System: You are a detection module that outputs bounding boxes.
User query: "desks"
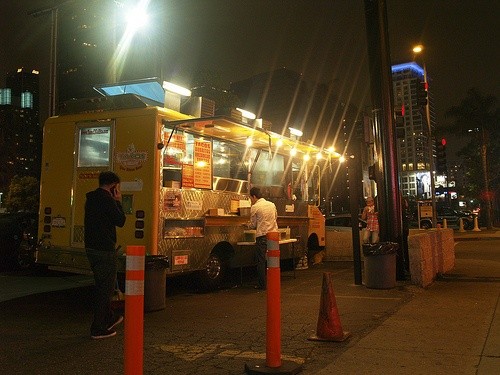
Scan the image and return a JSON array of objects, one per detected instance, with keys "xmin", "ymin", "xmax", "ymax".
[{"xmin": 237, "ymin": 237, "xmax": 298, "ymax": 289}]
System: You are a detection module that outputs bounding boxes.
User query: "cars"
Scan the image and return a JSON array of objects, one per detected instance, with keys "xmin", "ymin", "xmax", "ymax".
[{"xmin": 420, "ymin": 206, "xmax": 481, "ymax": 230}]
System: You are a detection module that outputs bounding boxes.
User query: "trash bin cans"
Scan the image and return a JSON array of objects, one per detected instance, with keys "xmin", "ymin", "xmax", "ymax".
[
  {"xmin": 118, "ymin": 254, "xmax": 169, "ymax": 312},
  {"xmin": 360, "ymin": 243, "xmax": 399, "ymax": 289}
]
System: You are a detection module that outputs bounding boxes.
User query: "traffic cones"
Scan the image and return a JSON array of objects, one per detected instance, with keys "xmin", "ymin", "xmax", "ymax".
[{"xmin": 306, "ymin": 271, "xmax": 352, "ymax": 342}]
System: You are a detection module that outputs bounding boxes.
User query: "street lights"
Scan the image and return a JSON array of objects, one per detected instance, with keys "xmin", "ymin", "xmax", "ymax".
[{"xmin": 404, "ymin": 44, "xmax": 424, "ymax": 230}]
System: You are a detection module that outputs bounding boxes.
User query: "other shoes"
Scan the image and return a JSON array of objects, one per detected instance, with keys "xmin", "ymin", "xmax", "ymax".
[
  {"xmin": 90, "ymin": 329, "xmax": 117, "ymax": 340},
  {"xmin": 107, "ymin": 314, "xmax": 124, "ymax": 331}
]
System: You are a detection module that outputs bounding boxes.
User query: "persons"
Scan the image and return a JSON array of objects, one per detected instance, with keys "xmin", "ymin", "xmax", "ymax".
[
  {"xmin": 360, "ymin": 198, "xmax": 379, "ymax": 243},
  {"xmin": 85, "ymin": 171, "xmax": 126, "ymax": 339},
  {"xmin": 242, "ymin": 187, "xmax": 279, "ymax": 290},
  {"xmin": 292, "ymin": 189, "xmax": 310, "ymax": 270}
]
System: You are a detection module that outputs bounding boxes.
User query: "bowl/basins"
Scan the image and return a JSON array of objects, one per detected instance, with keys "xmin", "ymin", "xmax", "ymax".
[
  {"xmin": 238, "ymin": 207, "xmax": 251, "ymax": 216},
  {"xmin": 244, "ymin": 230, "xmax": 256, "ymax": 242}
]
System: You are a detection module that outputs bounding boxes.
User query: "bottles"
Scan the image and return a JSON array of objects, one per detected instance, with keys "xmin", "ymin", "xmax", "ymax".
[{"xmin": 286, "ymin": 226, "xmax": 290, "ymax": 240}]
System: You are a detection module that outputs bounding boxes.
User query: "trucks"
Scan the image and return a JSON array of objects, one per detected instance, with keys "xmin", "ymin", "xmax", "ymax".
[{"xmin": 31, "ymin": 75, "xmax": 343, "ymax": 294}]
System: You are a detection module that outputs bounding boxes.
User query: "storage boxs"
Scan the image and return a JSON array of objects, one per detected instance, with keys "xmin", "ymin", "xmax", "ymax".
[
  {"xmin": 239, "ymin": 208, "xmax": 251, "ymax": 217},
  {"xmin": 244, "ymin": 230, "xmax": 256, "ymax": 242}
]
